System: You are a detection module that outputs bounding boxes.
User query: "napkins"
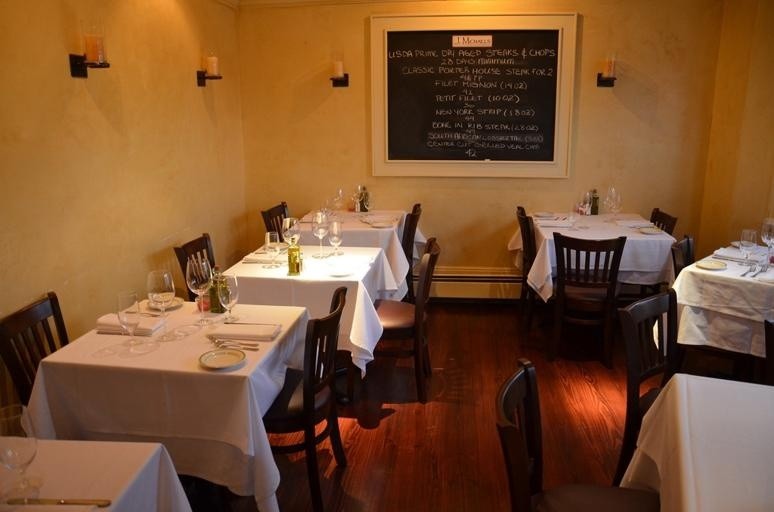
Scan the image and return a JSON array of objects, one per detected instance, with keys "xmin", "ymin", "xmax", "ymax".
[
  {"xmin": 616, "ymin": 221, "xmax": 650, "ymax": 228},
  {"xmin": 539, "ymin": 220, "xmax": 570, "ymax": 227},
  {"xmin": 362, "ymin": 215, "xmax": 388, "ymax": 223},
  {"xmin": 712, "ymin": 248, "xmax": 759, "ymax": 264},
  {"xmin": 97, "ymin": 313, "xmax": 164, "ymax": 336},
  {"xmin": 206, "ymin": 323, "xmax": 281, "ymax": 341},
  {"xmin": 242, "ymin": 254, "xmax": 274, "ymax": 264},
  {"xmin": 300, "ymin": 217, "xmax": 312, "ymax": 223}
]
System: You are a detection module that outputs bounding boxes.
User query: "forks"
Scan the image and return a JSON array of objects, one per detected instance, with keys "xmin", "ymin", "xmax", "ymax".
[
  {"xmin": 206, "ymin": 333, "xmax": 260, "ymax": 352},
  {"xmin": 740, "ymin": 264, "xmax": 768, "ymax": 279}
]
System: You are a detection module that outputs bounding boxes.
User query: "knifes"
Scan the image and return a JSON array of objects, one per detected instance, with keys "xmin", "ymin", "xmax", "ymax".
[{"xmin": 7, "ymin": 498, "xmax": 110, "ymax": 507}]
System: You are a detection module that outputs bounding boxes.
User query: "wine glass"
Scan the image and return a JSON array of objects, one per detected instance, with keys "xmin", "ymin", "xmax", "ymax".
[
  {"xmin": 740, "ymin": 219, "xmax": 774, "ymax": 268},
  {"xmin": 260, "ymin": 210, "xmax": 342, "ymax": 267},
  {"xmin": 115, "ymin": 291, "xmax": 145, "ymax": 347},
  {"xmin": 0, "ymin": 405, "xmax": 39, "ymax": 504},
  {"xmin": 603, "ymin": 187, "xmax": 623, "ymax": 224},
  {"xmin": 186, "ymin": 258, "xmax": 240, "ymax": 327},
  {"xmin": 324, "ymin": 186, "xmax": 376, "ymax": 222},
  {"xmin": 147, "ymin": 269, "xmax": 180, "ymax": 341}
]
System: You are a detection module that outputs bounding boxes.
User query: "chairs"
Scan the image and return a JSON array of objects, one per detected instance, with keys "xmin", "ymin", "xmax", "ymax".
[
  {"xmin": 611, "ymin": 288, "xmax": 682, "ymax": 487},
  {"xmin": 516, "ymin": 206, "xmax": 538, "ymax": 332},
  {"xmin": 261, "ymin": 202, "xmax": 289, "ymax": 243},
  {"xmin": 764, "ymin": 320, "xmax": 774, "ymax": 385},
  {"xmin": 552, "ymin": 232, "xmax": 627, "ymax": 359},
  {"xmin": 640, "ymin": 208, "xmax": 677, "ymax": 298},
  {"xmin": 671, "ymin": 235, "xmax": 694, "ymax": 280},
  {"xmin": 348, "ymin": 236, "xmax": 440, "ymax": 405},
  {"xmin": 261, "ymin": 286, "xmax": 348, "ymax": 512},
  {"xmin": 173, "ymin": 233, "xmax": 215, "ymax": 302},
  {"xmin": 493, "ymin": 358, "xmax": 661, "ymax": 512},
  {"xmin": 401, "ymin": 203, "xmax": 422, "ymax": 305},
  {"xmin": 1, "ymin": 291, "xmax": 69, "ymax": 408}
]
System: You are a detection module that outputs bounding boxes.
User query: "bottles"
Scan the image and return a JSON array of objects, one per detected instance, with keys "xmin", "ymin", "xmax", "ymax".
[
  {"xmin": 576, "ymin": 189, "xmax": 598, "ymax": 215},
  {"xmin": 287, "ymin": 238, "xmax": 301, "ymax": 275}
]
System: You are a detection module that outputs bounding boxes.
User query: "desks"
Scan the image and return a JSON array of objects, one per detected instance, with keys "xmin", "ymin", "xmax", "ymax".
[
  {"xmin": 40, "ymin": 298, "xmax": 308, "ymax": 512},
  {"xmin": 0, "ymin": 436, "xmax": 191, "ymax": 512},
  {"xmin": 222, "ymin": 245, "xmax": 383, "ymax": 353},
  {"xmin": 682, "ymin": 245, "xmax": 773, "ymax": 358},
  {"xmin": 668, "ymin": 374, "xmax": 774, "ymax": 512},
  {"xmin": 290, "ymin": 209, "xmax": 408, "ymax": 302},
  {"xmin": 528, "ymin": 213, "xmax": 676, "ymax": 347}
]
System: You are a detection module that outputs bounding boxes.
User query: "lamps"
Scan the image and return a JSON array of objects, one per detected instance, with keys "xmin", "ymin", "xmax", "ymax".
[
  {"xmin": 595, "ymin": 56, "xmax": 616, "ymax": 88},
  {"xmin": 68, "ymin": 33, "xmax": 108, "ymax": 79},
  {"xmin": 194, "ymin": 56, "xmax": 222, "ymax": 87},
  {"xmin": 330, "ymin": 60, "xmax": 349, "ymax": 88}
]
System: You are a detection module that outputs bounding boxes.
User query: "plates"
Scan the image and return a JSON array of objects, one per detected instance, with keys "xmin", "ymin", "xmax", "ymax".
[
  {"xmin": 641, "ymin": 228, "xmax": 663, "ymax": 235},
  {"xmin": 695, "ymin": 259, "xmax": 727, "ymax": 271},
  {"xmin": 533, "ymin": 211, "xmax": 555, "ymax": 219},
  {"xmin": 731, "ymin": 241, "xmax": 754, "ymax": 248},
  {"xmin": 370, "ymin": 222, "xmax": 394, "ymax": 228},
  {"xmin": 198, "ymin": 348, "xmax": 246, "ymax": 371}
]
